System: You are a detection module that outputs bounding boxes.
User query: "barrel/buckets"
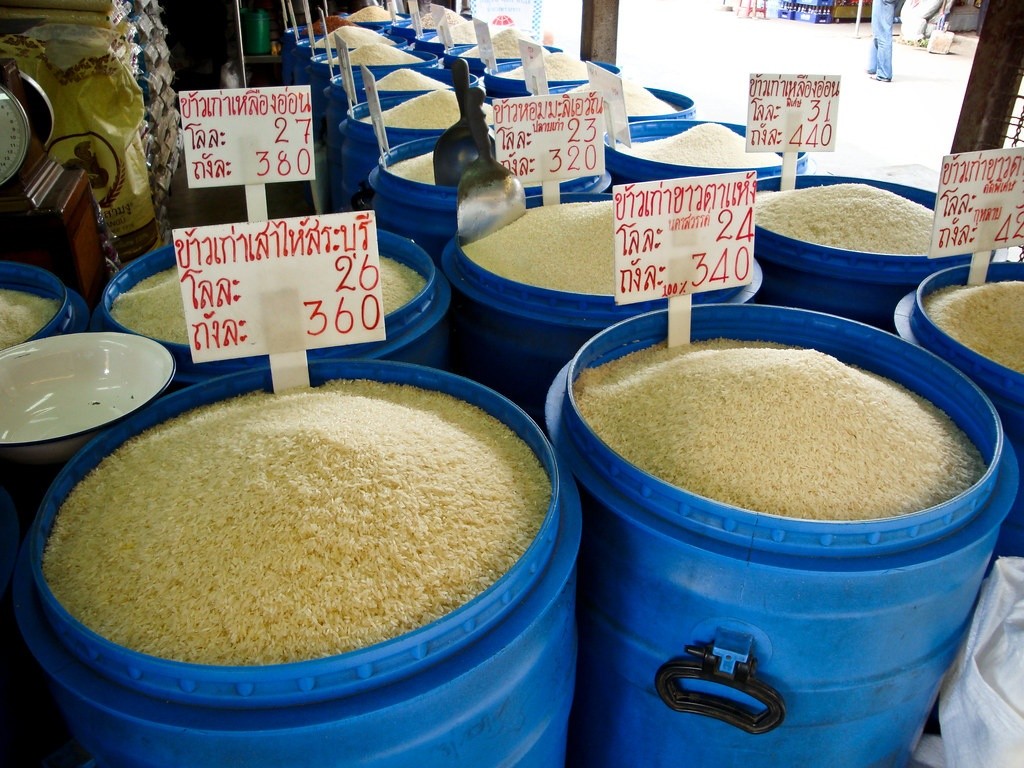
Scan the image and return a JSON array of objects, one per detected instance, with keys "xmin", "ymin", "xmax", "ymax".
[{"xmin": 0, "ymin": 12, "xmax": 1024, "ymax": 767}]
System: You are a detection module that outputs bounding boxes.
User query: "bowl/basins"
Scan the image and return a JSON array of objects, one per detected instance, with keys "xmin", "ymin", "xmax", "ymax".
[{"xmin": 1, "ymin": 331, "xmax": 177, "ymax": 466}]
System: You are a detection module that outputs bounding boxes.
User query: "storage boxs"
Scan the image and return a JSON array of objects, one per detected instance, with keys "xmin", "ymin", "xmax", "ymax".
[{"xmin": 778, "ymin": 0, "xmax": 835, "ymax": 23}]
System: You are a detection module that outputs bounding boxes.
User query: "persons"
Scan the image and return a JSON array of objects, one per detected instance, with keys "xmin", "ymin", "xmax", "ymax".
[{"xmin": 865, "ymin": 0, "xmax": 895, "ymax": 83}]
